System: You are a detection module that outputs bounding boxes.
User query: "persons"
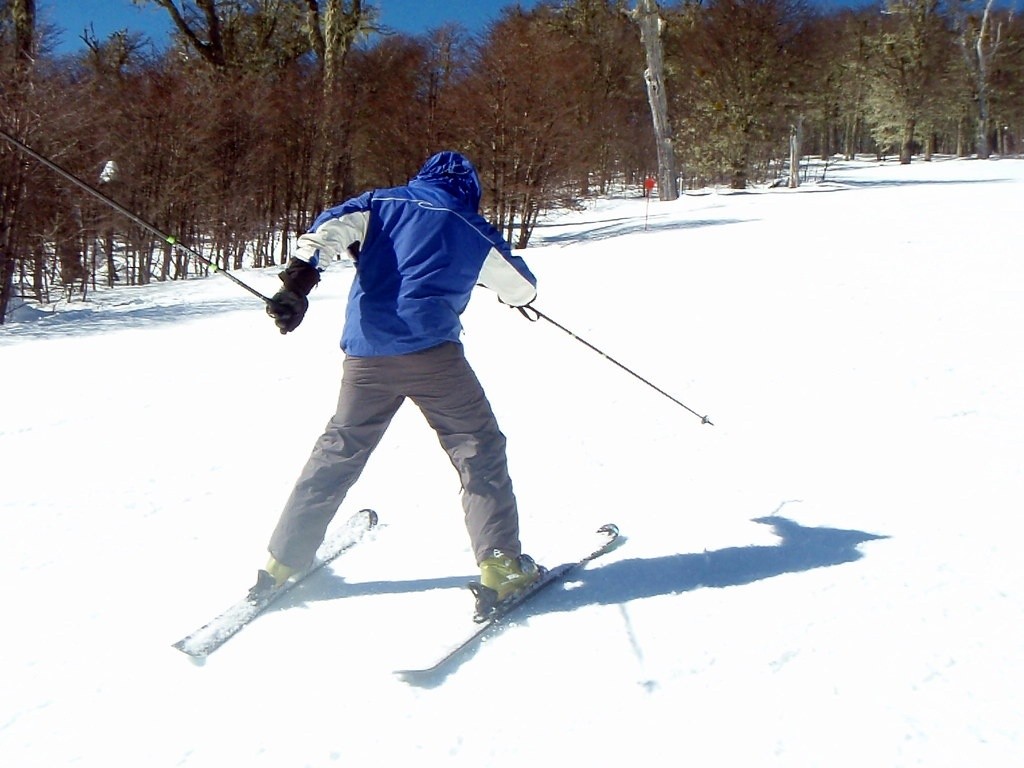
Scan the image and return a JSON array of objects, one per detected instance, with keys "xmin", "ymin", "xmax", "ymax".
[{"xmin": 247, "ymin": 150, "xmax": 543, "ymax": 623}]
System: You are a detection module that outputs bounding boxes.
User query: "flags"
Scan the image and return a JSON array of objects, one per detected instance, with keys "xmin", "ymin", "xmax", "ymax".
[{"xmin": 645, "ymin": 178, "xmax": 655, "ymax": 189}]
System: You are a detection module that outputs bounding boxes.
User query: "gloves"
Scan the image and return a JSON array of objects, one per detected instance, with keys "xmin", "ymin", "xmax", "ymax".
[{"xmin": 266, "ymin": 256, "xmax": 321, "ymax": 335}]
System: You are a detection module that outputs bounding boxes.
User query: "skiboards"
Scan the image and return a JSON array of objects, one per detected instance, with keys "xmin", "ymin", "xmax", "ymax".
[
  {"xmin": 174, "ymin": 507, "xmax": 380, "ymax": 664},
  {"xmin": 404, "ymin": 522, "xmax": 618, "ymax": 674}
]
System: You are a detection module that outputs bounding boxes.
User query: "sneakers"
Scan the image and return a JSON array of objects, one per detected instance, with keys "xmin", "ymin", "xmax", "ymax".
[
  {"xmin": 480, "ymin": 549, "xmax": 547, "ymax": 601},
  {"xmin": 267, "ymin": 555, "xmax": 313, "ymax": 587}
]
